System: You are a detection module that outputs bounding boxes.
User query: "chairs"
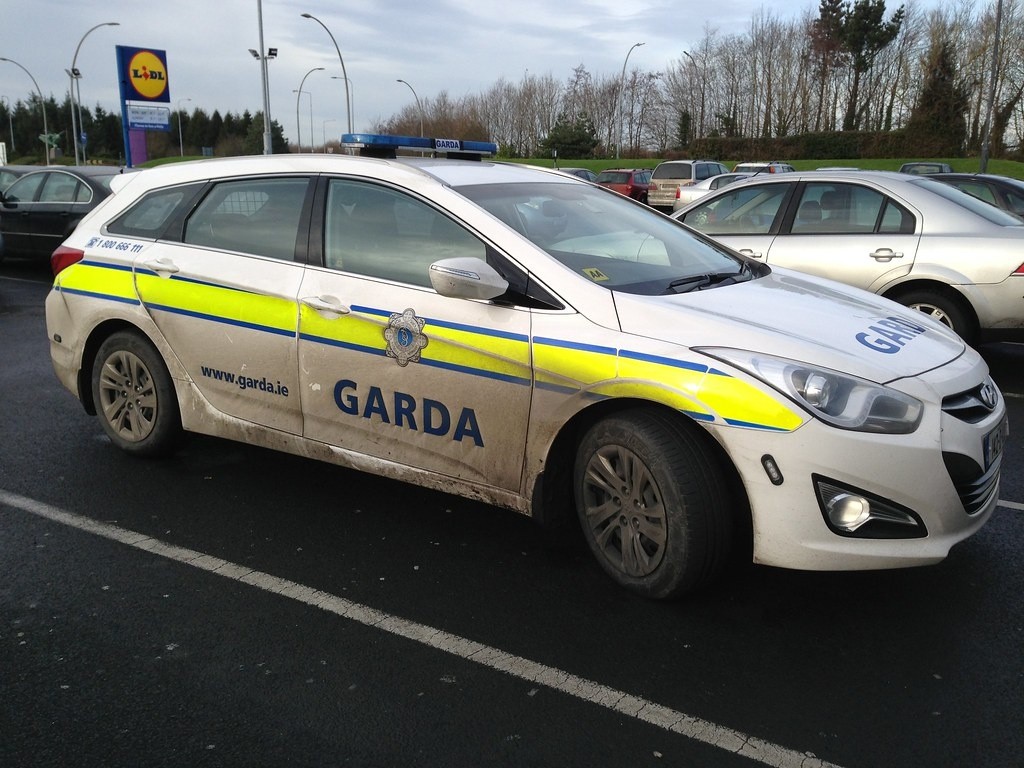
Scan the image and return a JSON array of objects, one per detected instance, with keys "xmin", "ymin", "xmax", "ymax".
[
  {"xmin": 792, "ymin": 200, "xmax": 823, "ymax": 232},
  {"xmin": 912, "ymin": 170, "xmax": 920, "ymax": 173},
  {"xmin": 927, "ymin": 169, "xmax": 935, "ymax": 173},
  {"xmin": 427, "ymin": 210, "xmax": 486, "ymax": 295},
  {"xmin": 345, "ymin": 195, "xmax": 402, "ymax": 278},
  {"xmin": 54, "ymin": 185, "xmax": 75, "ymax": 202},
  {"xmin": 820, "ymin": 190, "xmax": 844, "ymax": 231}
]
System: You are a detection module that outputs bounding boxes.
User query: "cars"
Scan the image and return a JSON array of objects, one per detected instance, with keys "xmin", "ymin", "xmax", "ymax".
[
  {"xmin": 43, "ymin": 146, "xmax": 1011, "ymax": 606},
  {"xmin": 544, "ymin": 167, "xmax": 1023, "ymax": 350},
  {"xmin": 520, "ymin": 158, "xmax": 1023, "ymax": 247},
  {"xmin": 0, "ymin": 165, "xmax": 176, "ymax": 279}
]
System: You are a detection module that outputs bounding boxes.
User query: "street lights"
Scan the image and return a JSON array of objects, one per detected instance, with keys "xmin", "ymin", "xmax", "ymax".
[
  {"xmin": 615, "ymin": 42, "xmax": 647, "ymax": 160},
  {"xmin": 397, "ymin": 78, "xmax": 425, "ymax": 157},
  {"xmin": 292, "ymin": 89, "xmax": 314, "ymax": 153},
  {"xmin": 68, "ymin": 22, "xmax": 119, "ymax": 167},
  {"xmin": 295, "ymin": 67, "xmax": 325, "ymax": 152},
  {"xmin": 330, "ymin": 76, "xmax": 355, "ymax": 134},
  {"xmin": 0, "ymin": 56, "xmax": 50, "ymax": 164},
  {"xmin": 300, "ymin": 13, "xmax": 351, "ymax": 134}
]
